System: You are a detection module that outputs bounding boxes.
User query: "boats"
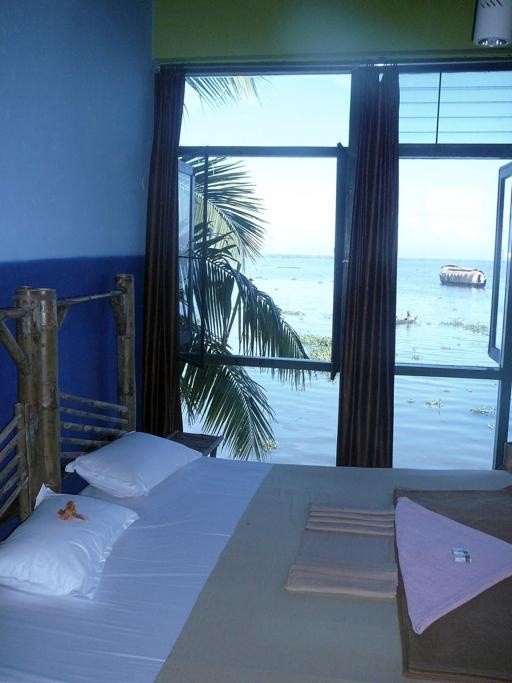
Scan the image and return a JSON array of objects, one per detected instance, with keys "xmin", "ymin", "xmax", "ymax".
[
  {"xmin": 396, "ymin": 311, "xmax": 417, "ymax": 324},
  {"xmin": 439, "ymin": 264, "xmax": 486, "ymax": 288}
]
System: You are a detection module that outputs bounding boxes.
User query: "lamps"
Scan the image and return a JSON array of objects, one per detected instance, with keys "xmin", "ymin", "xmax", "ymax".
[{"xmin": 471, "ymin": 0, "xmax": 512, "ymax": 50}]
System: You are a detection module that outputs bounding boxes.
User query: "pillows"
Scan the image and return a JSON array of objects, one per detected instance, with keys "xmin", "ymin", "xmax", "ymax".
[{"xmin": 0, "ymin": 430, "xmax": 202, "ymax": 596}]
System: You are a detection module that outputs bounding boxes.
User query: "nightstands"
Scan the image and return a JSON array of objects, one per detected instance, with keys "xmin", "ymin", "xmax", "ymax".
[{"xmin": 166, "ymin": 430, "xmax": 225, "ymax": 459}]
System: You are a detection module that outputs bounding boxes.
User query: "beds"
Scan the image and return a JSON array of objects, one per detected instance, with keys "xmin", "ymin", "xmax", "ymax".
[{"xmin": 0, "ymin": 274, "xmax": 512, "ymax": 683}]
